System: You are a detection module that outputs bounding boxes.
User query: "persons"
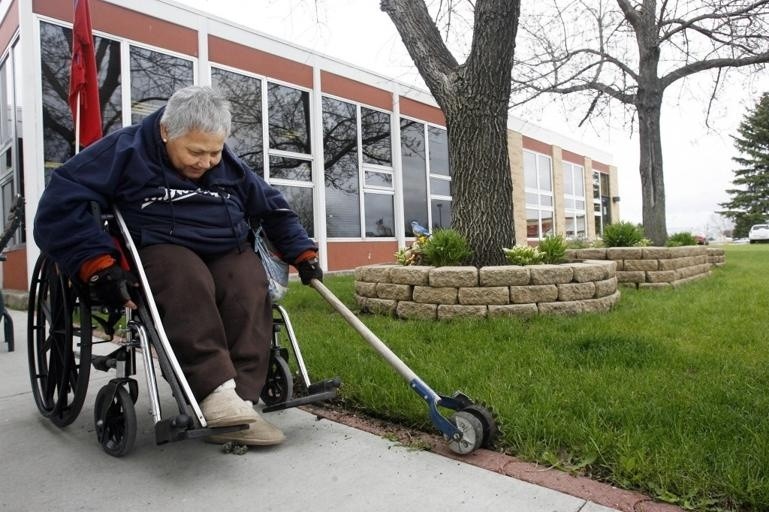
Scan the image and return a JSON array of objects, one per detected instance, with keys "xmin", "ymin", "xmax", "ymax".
[{"xmin": 32, "ymin": 84, "xmax": 325, "ymax": 448}]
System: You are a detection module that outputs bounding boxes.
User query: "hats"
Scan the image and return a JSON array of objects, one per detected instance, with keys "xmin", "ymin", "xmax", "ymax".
[{"xmin": 749, "ymin": 225, "xmax": 769, "ymax": 244}]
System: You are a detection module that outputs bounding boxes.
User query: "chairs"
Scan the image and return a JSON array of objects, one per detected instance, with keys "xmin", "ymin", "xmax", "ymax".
[
  {"xmin": 206, "ymin": 402, "xmax": 287, "ymax": 448},
  {"xmin": 192, "ymin": 379, "xmax": 259, "ymax": 430}
]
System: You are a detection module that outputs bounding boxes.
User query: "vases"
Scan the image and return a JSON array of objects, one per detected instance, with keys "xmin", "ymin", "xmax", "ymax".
[{"xmin": 501, "ymin": 242, "xmax": 547, "ymax": 265}]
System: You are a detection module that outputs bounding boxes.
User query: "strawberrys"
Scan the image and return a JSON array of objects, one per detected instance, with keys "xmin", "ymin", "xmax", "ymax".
[{"xmin": 28, "ymin": 206, "xmax": 341, "ymax": 457}]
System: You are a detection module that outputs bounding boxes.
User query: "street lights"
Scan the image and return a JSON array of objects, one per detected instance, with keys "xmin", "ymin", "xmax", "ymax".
[{"xmin": 253, "ymin": 225, "xmax": 290, "ymax": 306}]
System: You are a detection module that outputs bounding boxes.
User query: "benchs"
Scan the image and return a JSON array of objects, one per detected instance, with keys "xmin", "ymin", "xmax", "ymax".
[{"xmin": 66, "ymin": 1, "xmax": 104, "ymax": 146}]
